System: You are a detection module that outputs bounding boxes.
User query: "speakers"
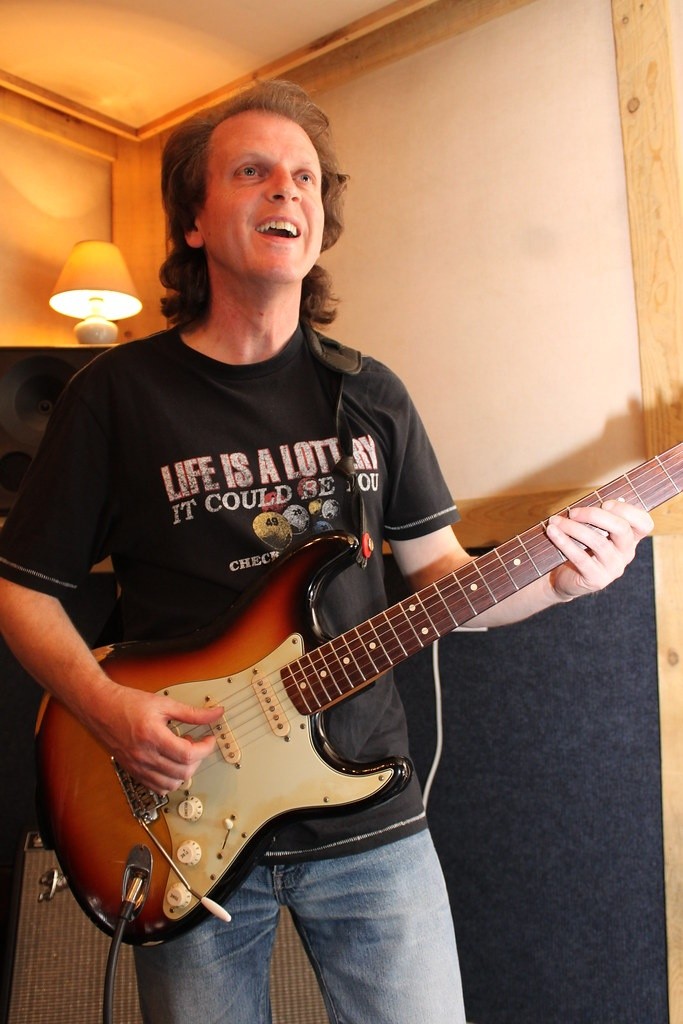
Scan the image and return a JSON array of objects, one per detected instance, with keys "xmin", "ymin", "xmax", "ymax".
[{"xmin": 0, "ymin": 829, "xmax": 333, "ymax": 1024}]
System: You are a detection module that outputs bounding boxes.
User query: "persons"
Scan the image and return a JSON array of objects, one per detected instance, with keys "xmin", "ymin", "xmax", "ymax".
[{"xmin": 0, "ymin": 79, "xmax": 655, "ymax": 1024}]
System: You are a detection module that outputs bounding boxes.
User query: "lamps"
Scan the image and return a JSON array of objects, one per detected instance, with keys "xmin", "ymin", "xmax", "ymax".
[{"xmin": 49, "ymin": 241, "xmax": 142, "ymax": 344}]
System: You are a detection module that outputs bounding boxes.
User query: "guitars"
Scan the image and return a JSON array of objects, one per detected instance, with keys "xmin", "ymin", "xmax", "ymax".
[{"xmin": 14, "ymin": 438, "xmax": 683, "ymax": 950}]
